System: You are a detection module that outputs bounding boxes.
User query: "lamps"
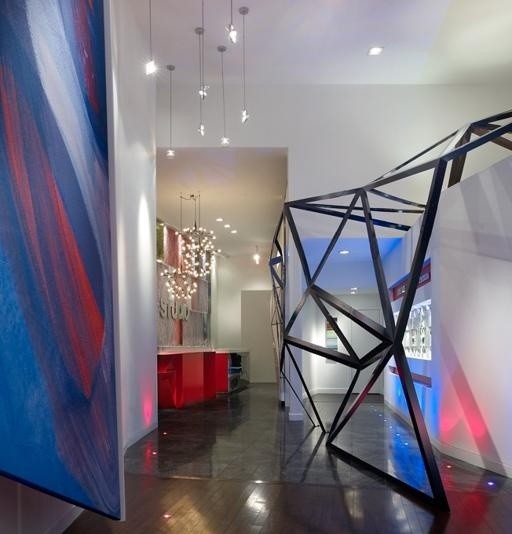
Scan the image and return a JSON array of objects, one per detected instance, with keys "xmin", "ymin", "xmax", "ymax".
[
  {"xmin": 148, "ymin": 0, "xmax": 250, "ymax": 158},
  {"xmin": 161, "ymin": 255, "xmax": 198, "ymax": 303},
  {"xmin": 178, "ymin": 192, "xmax": 221, "ymax": 278}
]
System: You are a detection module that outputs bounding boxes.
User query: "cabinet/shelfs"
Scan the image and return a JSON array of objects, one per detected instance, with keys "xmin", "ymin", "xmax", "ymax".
[{"xmin": 225, "ymin": 351, "xmax": 250, "ymax": 394}]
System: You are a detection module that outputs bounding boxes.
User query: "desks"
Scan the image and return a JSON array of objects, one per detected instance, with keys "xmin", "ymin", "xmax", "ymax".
[{"xmin": 157, "ymin": 350, "xmax": 229, "ymax": 410}]
